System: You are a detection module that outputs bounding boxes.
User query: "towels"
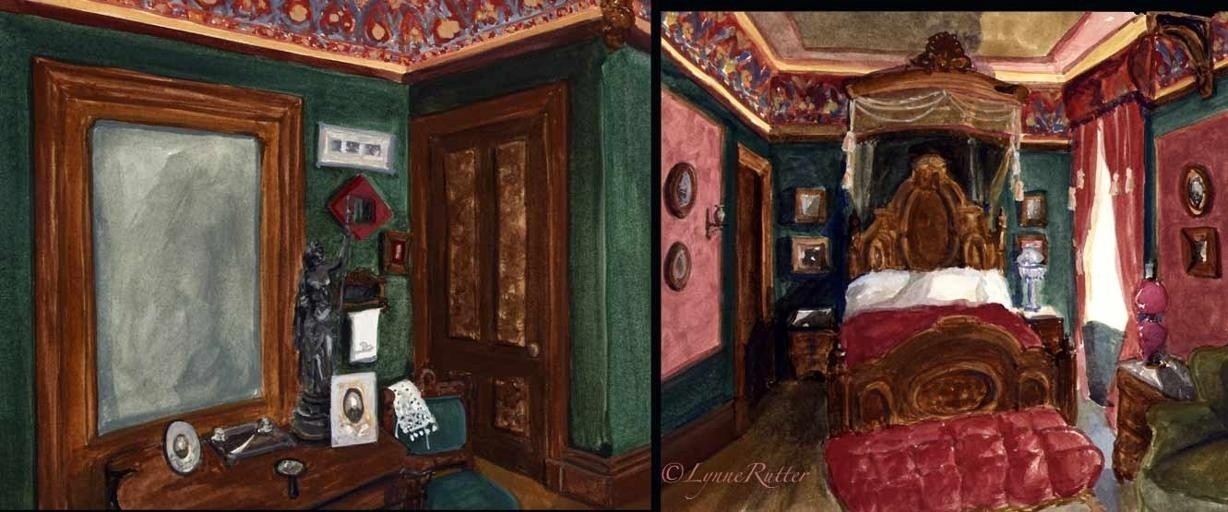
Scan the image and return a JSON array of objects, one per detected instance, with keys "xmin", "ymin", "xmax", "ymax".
[{"xmin": 346, "ymin": 307, "xmax": 383, "ymax": 364}]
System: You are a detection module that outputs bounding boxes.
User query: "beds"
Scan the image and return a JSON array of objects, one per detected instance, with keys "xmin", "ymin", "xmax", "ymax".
[{"xmin": 825, "ymin": 153, "xmax": 1078, "ymax": 438}]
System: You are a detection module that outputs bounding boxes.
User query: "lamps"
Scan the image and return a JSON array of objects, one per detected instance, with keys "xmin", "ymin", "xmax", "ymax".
[
  {"xmin": 706, "ymin": 204, "xmax": 727, "ymax": 240},
  {"xmin": 1016, "ymin": 243, "xmax": 1046, "ymax": 312}
]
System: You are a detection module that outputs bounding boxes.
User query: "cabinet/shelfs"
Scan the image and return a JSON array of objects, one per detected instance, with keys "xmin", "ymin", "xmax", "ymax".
[{"xmin": 1113, "ymin": 355, "xmax": 1198, "ymax": 486}]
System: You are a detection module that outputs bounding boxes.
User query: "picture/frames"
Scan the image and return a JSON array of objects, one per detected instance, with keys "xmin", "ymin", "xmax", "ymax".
[
  {"xmin": 664, "ymin": 162, "xmax": 698, "ymax": 292},
  {"xmin": 315, "ymin": 121, "xmax": 416, "ymax": 276},
  {"xmin": 1013, "ymin": 189, "xmax": 1049, "ymax": 264},
  {"xmin": 1179, "ymin": 163, "xmax": 1222, "ymax": 279},
  {"xmin": 791, "ymin": 187, "xmax": 830, "ymax": 275},
  {"xmin": 330, "ymin": 370, "xmax": 379, "ymax": 449}
]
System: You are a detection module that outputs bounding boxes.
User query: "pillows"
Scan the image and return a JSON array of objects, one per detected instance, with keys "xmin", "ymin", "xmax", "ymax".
[
  {"xmin": 845, "ymin": 269, "xmax": 910, "ymax": 306},
  {"xmin": 926, "ymin": 273, "xmax": 980, "ymax": 303}
]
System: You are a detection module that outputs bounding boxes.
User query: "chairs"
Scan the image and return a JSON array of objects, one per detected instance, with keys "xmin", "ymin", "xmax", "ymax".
[
  {"xmin": 384, "ymin": 369, "xmax": 521, "ymax": 510},
  {"xmin": 1133, "ymin": 344, "xmax": 1228, "ymax": 512}
]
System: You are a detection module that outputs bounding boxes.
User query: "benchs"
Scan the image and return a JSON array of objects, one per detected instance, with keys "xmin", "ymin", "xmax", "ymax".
[{"xmin": 816, "ymin": 406, "xmax": 1106, "ymax": 512}]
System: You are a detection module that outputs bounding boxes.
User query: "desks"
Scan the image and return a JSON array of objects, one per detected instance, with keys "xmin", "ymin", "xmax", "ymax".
[{"xmin": 105, "ymin": 423, "xmax": 432, "ymax": 510}]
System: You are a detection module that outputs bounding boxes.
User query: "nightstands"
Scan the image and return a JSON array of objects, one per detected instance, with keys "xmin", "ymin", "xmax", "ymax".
[
  {"xmin": 786, "ymin": 306, "xmax": 841, "ymax": 380},
  {"xmin": 1012, "ymin": 304, "xmax": 1064, "ymax": 347}
]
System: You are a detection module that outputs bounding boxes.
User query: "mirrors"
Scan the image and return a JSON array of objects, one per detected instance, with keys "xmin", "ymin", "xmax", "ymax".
[{"xmin": 35, "ymin": 53, "xmax": 304, "ymax": 449}]
